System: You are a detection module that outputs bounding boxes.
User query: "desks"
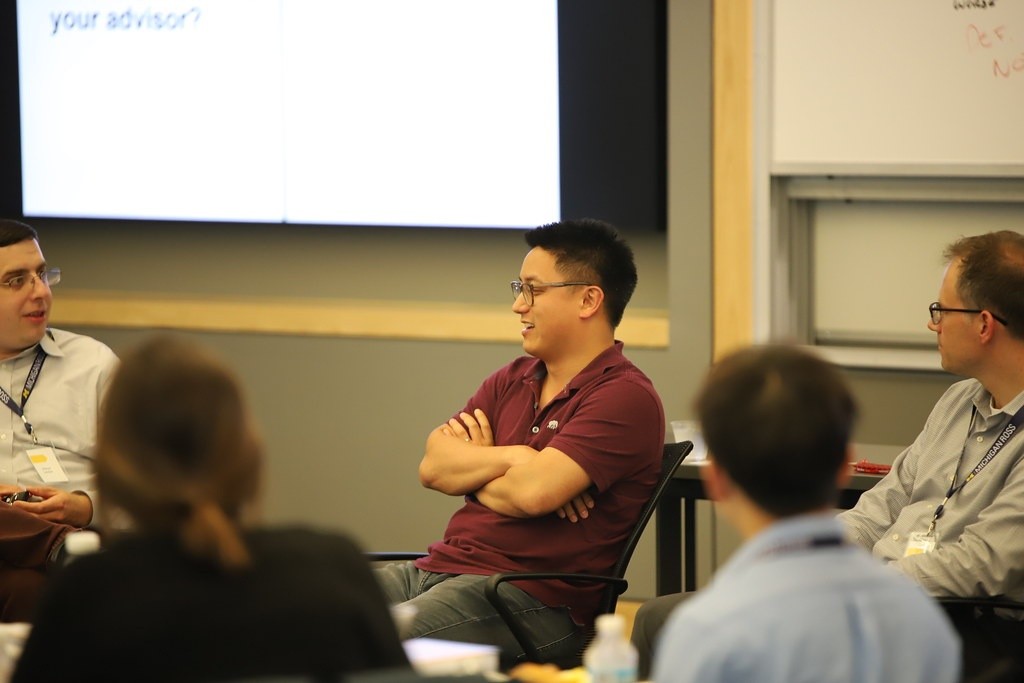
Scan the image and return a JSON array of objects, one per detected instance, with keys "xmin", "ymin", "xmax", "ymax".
[{"xmin": 657, "ymin": 419, "xmax": 909, "ymax": 620}]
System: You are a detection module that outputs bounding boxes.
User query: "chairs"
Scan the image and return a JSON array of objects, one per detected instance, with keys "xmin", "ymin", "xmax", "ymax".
[
  {"xmin": 356, "ymin": 440, "xmax": 693, "ymax": 671},
  {"xmin": 934, "ymin": 596, "xmax": 1024, "ymax": 683}
]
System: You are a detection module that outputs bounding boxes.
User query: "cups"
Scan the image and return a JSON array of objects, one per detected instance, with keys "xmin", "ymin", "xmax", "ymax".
[{"xmin": 671, "ymin": 420, "xmax": 708, "ymax": 463}]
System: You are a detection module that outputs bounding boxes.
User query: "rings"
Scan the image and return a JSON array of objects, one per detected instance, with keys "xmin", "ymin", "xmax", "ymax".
[{"xmin": 465, "ymin": 437, "xmax": 471, "ymax": 442}]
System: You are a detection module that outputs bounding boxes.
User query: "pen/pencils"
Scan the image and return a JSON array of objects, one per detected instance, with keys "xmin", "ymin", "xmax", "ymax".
[{"xmin": 848, "ymin": 462, "xmax": 892, "ymax": 474}]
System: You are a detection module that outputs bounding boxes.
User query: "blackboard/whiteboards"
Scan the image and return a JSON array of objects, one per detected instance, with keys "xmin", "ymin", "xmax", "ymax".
[{"xmin": 771, "ymin": 2, "xmax": 1023, "ymax": 355}]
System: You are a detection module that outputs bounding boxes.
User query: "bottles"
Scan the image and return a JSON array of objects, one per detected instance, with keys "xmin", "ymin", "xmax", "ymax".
[
  {"xmin": 584, "ymin": 614, "xmax": 640, "ymax": 683},
  {"xmin": 64, "ymin": 530, "xmax": 100, "ymax": 566}
]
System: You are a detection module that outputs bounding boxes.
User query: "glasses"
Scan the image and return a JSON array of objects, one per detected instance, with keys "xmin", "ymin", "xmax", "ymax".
[
  {"xmin": 511, "ymin": 281, "xmax": 607, "ymax": 306},
  {"xmin": 929, "ymin": 302, "xmax": 1008, "ymax": 326},
  {"xmin": 0, "ymin": 267, "xmax": 61, "ymax": 292}
]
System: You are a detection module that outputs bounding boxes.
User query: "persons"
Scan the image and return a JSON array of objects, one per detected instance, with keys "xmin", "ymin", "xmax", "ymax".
[
  {"xmin": 373, "ymin": 223, "xmax": 664, "ymax": 670},
  {"xmin": 8, "ymin": 336, "xmax": 414, "ymax": 683},
  {"xmin": 651, "ymin": 344, "xmax": 965, "ymax": 683},
  {"xmin": 0, "ymin": 220, "xmax": 128, "ymax": 624},
  {"xmin": 629, "ymin": 230, "xmax": 1024, "ymax": 683}
]
